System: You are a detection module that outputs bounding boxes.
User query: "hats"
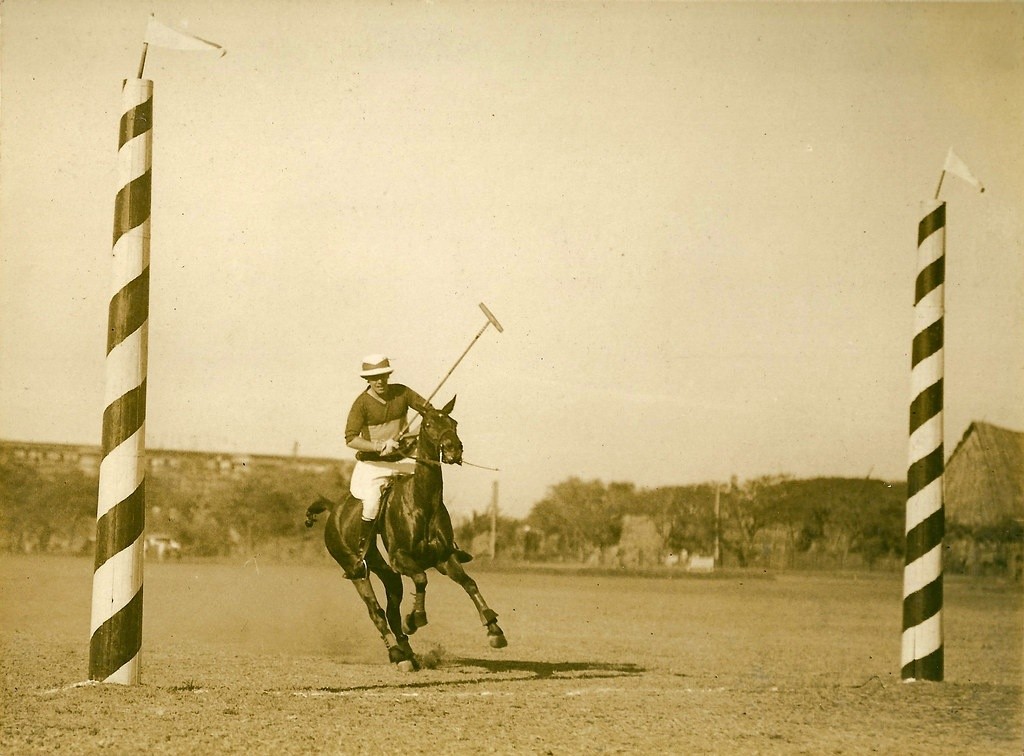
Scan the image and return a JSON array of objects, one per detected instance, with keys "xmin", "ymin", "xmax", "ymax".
[{"xmin": 360, "ymin": 354, "xmax": 393, "ymax": 376}]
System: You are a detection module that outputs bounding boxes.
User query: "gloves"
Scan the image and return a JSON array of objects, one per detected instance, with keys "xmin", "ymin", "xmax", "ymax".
[{"xmin": 378, "ymin": 438, "xmax": 400, "ymax": 456}]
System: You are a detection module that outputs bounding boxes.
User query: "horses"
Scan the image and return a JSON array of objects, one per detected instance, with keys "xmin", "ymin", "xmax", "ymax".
[{"xmin": 305, "ymin": 394, "xmax": 508, "ymax": 674}]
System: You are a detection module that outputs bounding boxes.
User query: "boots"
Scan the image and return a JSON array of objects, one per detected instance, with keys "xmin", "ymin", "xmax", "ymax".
[
  {"xmin": 341, "ymin": 515, "xmax": 375, "ymax": 580},
  {"xmin": 455, "ymin": 548, "xmax": 473, "ymax": 563}
]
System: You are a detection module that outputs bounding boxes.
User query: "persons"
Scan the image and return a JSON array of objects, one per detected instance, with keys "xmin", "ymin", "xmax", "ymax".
[{"xmin": 346, "ymin": 355, "xmax": 446, "ymax": 580}]
